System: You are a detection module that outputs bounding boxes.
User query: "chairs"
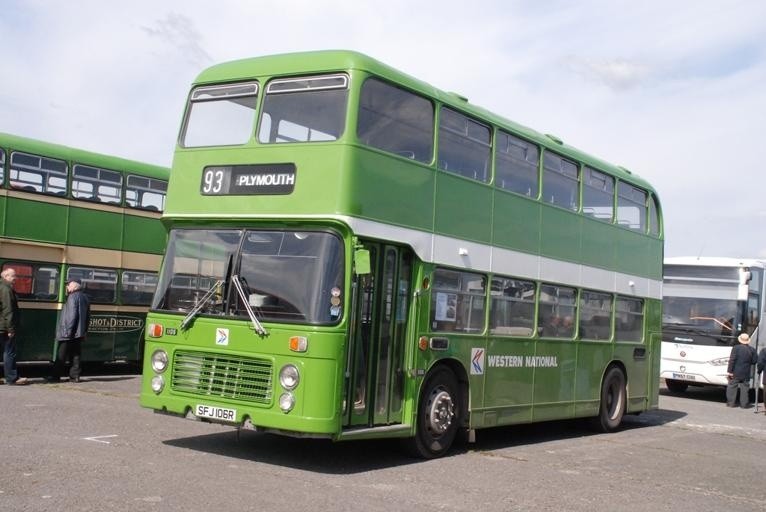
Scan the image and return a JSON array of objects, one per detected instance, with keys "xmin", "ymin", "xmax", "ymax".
[{"xmin": 389, "ymin": 149, "xmax": 645, "ymax": 234}]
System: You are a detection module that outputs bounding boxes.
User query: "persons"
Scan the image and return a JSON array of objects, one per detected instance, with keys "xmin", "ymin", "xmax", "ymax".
[
  {"xmin": 0, "ymin": 268, "xmax": 28, "ymax": 385},
  {"xmin": 44, "ymin": 278, "xmax": 91, "ymax": 383},
  {"xmin": 726, "ymin": 333, "xmax": 758, "ymax": 409},
  {"xmin": 558, "ymin": 316, "xmax": 574, "ymax": 337},
  {"xmin": 757, "ymin": 348, "xmax": 766, "ymax": 411},
  {"xmin": 542, "ymin": 312, "xmax": 561, "ymax": 337}
]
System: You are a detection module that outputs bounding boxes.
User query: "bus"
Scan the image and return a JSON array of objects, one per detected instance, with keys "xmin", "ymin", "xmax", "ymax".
[
  {"xmin": 659, "ymin": 257, "xmax": 766, "ymax": 401},
  {"xmin": 138, "ymin": 52, "xmax": 668, "ymax": 455},
  {"xmin": 0, "ymin": 132, "xmax": 174, "ymax": 379}
]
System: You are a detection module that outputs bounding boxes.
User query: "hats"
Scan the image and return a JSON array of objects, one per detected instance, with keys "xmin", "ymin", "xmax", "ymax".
[
  {"xmin": 738, "ymin": 333, "xmax": 750, "ymax": 345},
  {"xmin": 65, "ymin": 278, "xmax": 81, "ymax": 284}
]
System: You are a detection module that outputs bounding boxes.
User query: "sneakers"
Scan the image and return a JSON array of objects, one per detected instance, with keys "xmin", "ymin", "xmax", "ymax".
[{"xmin": 7, "ymin": 377, "xmax": 27, "ymax": 385}]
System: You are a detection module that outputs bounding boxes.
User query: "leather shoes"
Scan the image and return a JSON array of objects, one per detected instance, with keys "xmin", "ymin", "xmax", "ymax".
[{"xmin": 43, "ymin": 376, "xmax": 79, "ymax": 383}]
